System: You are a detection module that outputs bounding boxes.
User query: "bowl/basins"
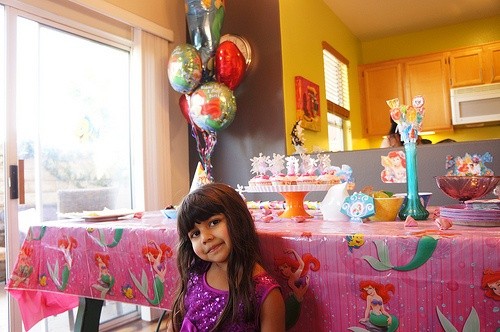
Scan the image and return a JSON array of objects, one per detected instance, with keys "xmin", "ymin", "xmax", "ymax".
[
  {"xmin": 432, "ymin": 175, "xmax": 500, "ymax": 204},
  {"xmin": 393, "ymin": 192, "xmax": 432, "ymax": 211},
  {"xmin": 368, "ymin": 196, "xmax": 405, "ymax": 222},
  {"xmin": 160, "ymin": 209, "xmax": 177, "ymax": 219}
]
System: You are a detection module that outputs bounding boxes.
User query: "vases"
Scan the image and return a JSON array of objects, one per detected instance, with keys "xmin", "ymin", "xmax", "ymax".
[{"xmin": 399, "ymin": 143, "xmax": 429, "ymax": 220}]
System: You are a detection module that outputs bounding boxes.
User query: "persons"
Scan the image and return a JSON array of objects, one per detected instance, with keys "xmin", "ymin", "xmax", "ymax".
[
  {"xmin": 165, "ymin": 182, "xmax": 286, "ymax": 332},
  {"xmin": 380, "ymin": 114, "xmax": 422, "ymax": 148}
]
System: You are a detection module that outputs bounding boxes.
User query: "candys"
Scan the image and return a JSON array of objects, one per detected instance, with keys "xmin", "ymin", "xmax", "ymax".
[{"xmin": 386, "ymin": 95, "xmax": 426, "ymax": 144}]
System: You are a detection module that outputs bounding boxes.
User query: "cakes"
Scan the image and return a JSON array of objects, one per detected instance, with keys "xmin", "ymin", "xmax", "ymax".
[{"xmin": 248, "ymin": 151, "xmax": 340, "ymax": 185}]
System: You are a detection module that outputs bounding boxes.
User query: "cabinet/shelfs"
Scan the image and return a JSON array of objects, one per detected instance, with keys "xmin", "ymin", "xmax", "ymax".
[
  {"xmin": 449, "ymin": 40, "xmax": 500, "ymax": 88},
  {"xmin": 358, "ymin": 51, "xmax": 454, "ymax": 138}
]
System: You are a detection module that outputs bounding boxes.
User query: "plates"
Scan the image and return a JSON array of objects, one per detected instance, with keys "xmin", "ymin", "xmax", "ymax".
[{"xmin": 80, "ymin": 213, "xmax": 130, "ymax": 222}]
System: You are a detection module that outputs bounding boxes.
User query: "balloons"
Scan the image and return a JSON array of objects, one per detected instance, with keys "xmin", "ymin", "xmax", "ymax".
[
  {"xmin": 216, "ymin": 42, "xmax": 248, "ymax": 89},
  {"xmin": 212, "ymin": 33, "xmax": 251, "ymax": 82},
  {"xmin": 190, "ymin": 81, "xmax": 237, "ymax": 133},
  {"xmin": 178, "ymin": 93, "xmax": 194, "ymax": 127},
  {"xmin": 184, "ymin": 0, "xmax": 225, "ymax": 82},
  {"xmin": 167, "ymin": 44, "xmax": 203, "ymax": 94}
]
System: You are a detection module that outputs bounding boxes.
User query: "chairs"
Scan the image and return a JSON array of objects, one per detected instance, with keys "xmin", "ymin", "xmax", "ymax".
[{"xmin": 44, "ymin": 188, "xmax": 124, "ymax": 332}]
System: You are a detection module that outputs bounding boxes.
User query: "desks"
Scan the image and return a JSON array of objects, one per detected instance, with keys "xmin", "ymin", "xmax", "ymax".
[{"xmin": 5, "ymin": 206, "xmax": 500, "ymax": 332}]
